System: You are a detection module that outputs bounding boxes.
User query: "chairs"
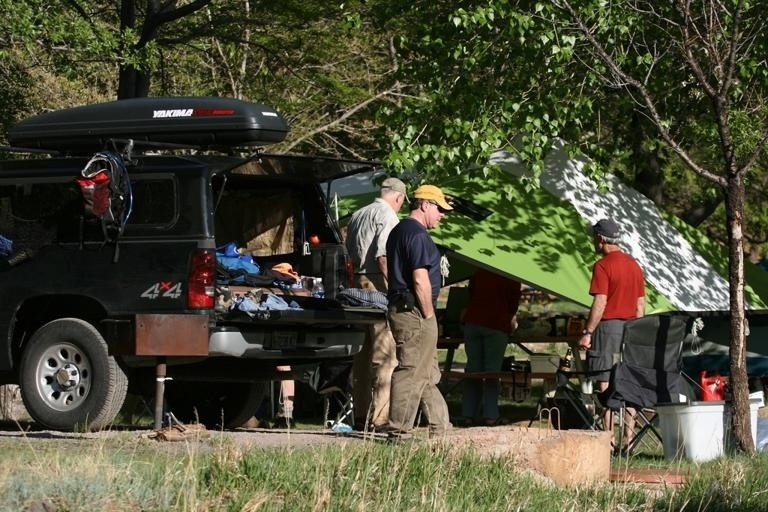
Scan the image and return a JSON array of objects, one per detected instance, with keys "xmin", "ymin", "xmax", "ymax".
[{"xmin": 603, "ymin": 310, "xmax": 691, "ymax": 460}]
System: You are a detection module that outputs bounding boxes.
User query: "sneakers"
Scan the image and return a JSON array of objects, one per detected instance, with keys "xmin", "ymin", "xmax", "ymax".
[
  {"xmin": 355, "ymin": 419, "xmax": 389, "ymax": 433},
  {"xmin": 465, "ymin": 416, "xmax": 508, "ymax": 426}
]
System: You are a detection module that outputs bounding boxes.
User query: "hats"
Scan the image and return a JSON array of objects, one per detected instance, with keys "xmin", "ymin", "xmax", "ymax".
[
  {"xmin": 594, "ymin": 220, "xmax": 620, "ymax": 238},
  {"xmin": 271, "ymin": 261, "xmax": 298, "ymax": 279},
  {"xmin": 412, "ymin": 185, "xmax": 454, "ymax": 211},
  {"xmin": 381, "ymin": 177, "xmax": 411, "ymax": 203}
]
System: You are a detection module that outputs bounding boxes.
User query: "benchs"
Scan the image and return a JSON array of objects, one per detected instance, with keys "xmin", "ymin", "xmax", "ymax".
[{"xmin": 388, "ymin": 336, "xmax": 617, "ymax": 428}]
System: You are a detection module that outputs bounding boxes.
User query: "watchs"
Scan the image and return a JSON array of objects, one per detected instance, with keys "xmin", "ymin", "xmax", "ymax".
[{"xmin": 581, "ymin": 328, "xmax": 593, "ymax": 336}]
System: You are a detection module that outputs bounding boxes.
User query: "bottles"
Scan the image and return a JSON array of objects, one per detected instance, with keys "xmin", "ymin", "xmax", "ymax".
[
  {"xmin": 312, "ymin": 277, "xmax": 325, "ymax": 299},
  {"xmin": 559, "ymin": 348, "xmax": 573, "ymax": 372}
]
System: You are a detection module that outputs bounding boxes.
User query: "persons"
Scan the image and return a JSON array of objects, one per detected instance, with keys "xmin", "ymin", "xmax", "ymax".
[
  {"xmin": 383, "ymin": 183, "xmax": 458, "ymax": 443},
  {"xmin": 573, "ymin": 218, "xmax": 647, "ymax": 454},
  {"xmin": 341, "ymin": 175, "xmax": 412, "ymax": 435},
  {"xmin": 461, "ymin": 265, "xmax": 523, "ymax": 426}
]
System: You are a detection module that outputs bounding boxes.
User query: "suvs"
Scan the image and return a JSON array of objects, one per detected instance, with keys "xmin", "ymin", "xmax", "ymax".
[{"xmin": 1, "ymin": 90, "xmax": 390, "ymax": 438}]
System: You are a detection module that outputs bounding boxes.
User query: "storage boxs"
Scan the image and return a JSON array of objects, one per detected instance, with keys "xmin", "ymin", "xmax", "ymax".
[{"xmin": 656, "ymin": 397, "xmax": 762, "ymax": 468}]
